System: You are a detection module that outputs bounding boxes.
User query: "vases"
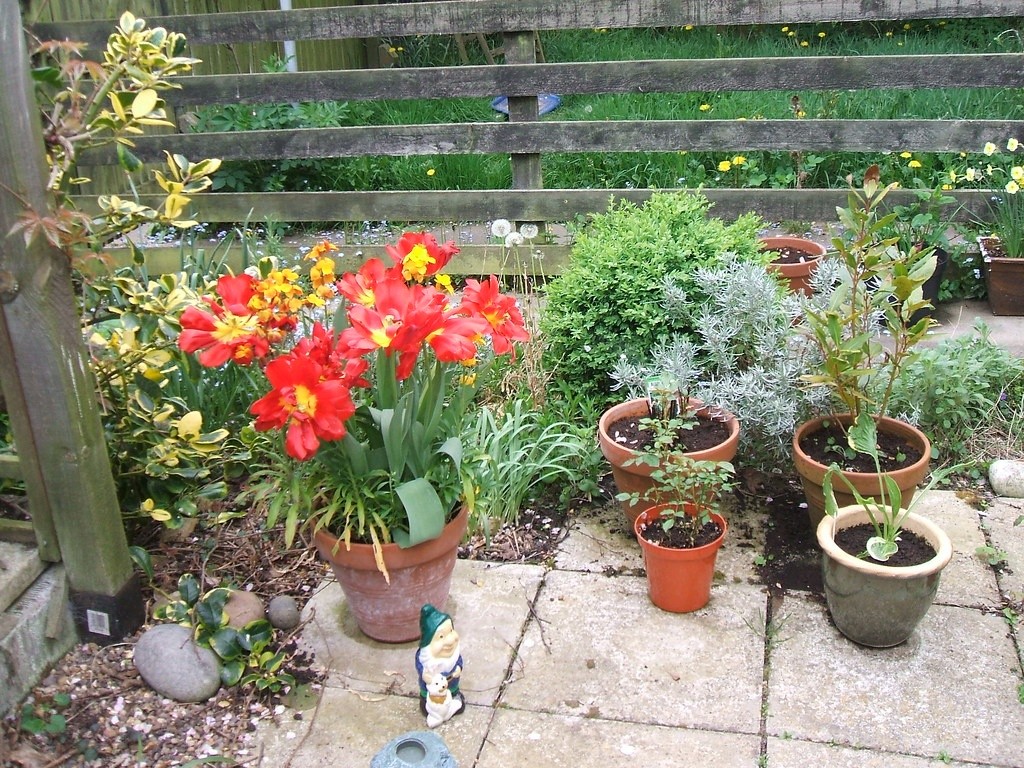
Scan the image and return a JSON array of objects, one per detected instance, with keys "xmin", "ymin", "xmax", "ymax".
[
  {"xmin": 975, "ymin": 237, "xmax": 1024, "ymax": 316},
  {"xmin": 856, "ymin": 166, "xmax": 949, "ymax": 330},
  {"xmin": 757, "ymin": 237, "xmax": 826, "ymax": 329},
  {"xmin": 310, "ymin": 490, "xmax": 469, "ymax": 643}
]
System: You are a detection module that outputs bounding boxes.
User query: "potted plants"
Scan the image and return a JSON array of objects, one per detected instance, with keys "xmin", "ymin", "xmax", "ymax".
[
  {"xmin": 791, "ymin": 166, "xmax": 938, "ymax": 528},
  {"xmin": 615, "ymin": 418, "xmax": 737, "ymax": 613},
  {"xmin": 814, "ymin": 413, "xmax": 953, "ymax": 648},
  {"xmin": 598, "ymin": 342, "xmax": 741, "ymax": 533}
]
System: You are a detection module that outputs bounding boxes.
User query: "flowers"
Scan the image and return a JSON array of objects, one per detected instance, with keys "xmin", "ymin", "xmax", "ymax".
[
  {"xmin": 177, "ymin": 234, "xmax": 532, "ymax": 487},
  {"xmin": 941, "ymin": 136, "xmax": 1024, "ymax": 257}
]
